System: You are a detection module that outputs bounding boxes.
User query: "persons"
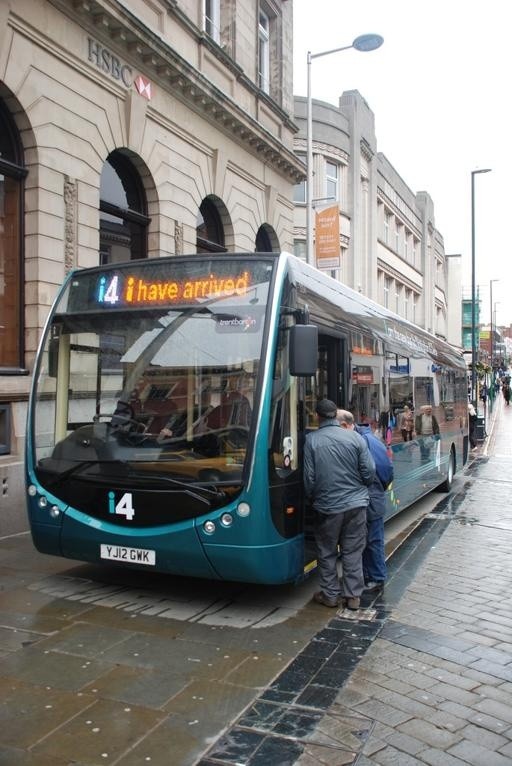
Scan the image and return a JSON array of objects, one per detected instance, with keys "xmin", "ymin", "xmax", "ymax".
[
  {"xmin": 474, "ymin": 353, "xmax": 512, "ymax": 408},
  {"xmin": 305, "ymin": 381, "xmax": 464, "ymax": 464},
  {"xmin": 301, "ymin": 397, "xmax": 379, "ymax": 609},
  {"xmin": 335, "ymin": 407, "xmax": 395, "ymax": 597}
]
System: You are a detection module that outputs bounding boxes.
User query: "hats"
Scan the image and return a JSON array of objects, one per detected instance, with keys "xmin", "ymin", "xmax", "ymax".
[{"xmin": 315, "ymin": 399, "xmax": 337, "ymax": 419}]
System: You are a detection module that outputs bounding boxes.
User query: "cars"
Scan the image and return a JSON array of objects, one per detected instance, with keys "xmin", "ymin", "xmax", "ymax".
[{"xmin": 130, "ymin": 448, "xmax": 241, "ymax": 485}]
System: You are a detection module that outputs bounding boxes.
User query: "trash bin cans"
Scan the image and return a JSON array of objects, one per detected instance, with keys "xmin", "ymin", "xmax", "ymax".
[{"xmin": 475, "ymin": 415, "xmax": 485, "ymax": 445}]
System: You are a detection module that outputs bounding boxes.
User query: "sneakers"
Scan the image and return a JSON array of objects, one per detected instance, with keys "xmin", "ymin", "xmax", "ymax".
[
  {"xmin": 363, "ymin": 581, "xmax": 384, "ymax": 594},
  {"xmin": 313, "ymin": 590, "xmax": 338, "ymax": 607},
  {"xmin": 346, "ymin": 597, "xmax": 360, "ymax": 610}
]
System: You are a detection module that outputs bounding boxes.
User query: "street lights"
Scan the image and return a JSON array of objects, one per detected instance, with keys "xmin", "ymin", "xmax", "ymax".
[
  {"xmin": 489, "ymin": 278, "xmax": 503, "ymax": 374},
  {"xmin": 301, "ymin": 28, "xmax": 388, "ymax": 275},
  {"xmin": 468, "ymin": 162, "xmax": 495, "ymax": 409},
  {"xmin": 494, "ymin": 301, "xmax": 502, "ymax": 354}
]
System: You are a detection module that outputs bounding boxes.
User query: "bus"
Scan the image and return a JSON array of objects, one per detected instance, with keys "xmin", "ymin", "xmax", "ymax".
[
  {"xmin": 20, "ymin": 249, "xmax": 478, "ymax": 590},
  {"xmin": 140, "ymin": 424, "xmax": 250, "ymax": 460}
]
